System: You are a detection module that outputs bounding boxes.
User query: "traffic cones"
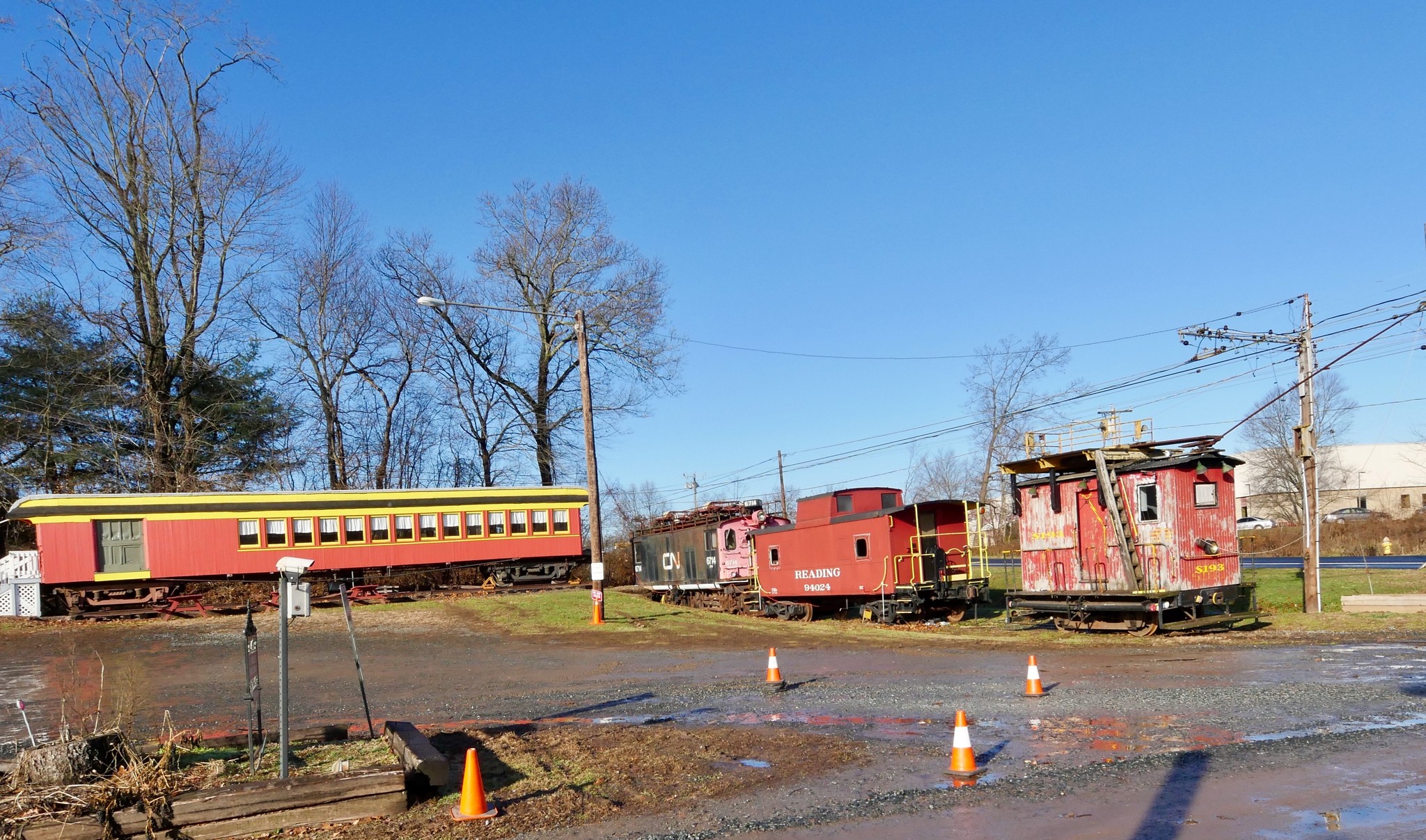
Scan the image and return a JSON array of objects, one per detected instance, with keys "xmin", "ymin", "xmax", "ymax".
[
  {"xmin": 587, "ymin": 597, "xmax": 606, "ymax": 625},
  {"xmin": 762, "ymin": 646, "xmax": 788, "ymax": 694},
  {"xmin": 941, "ymin": 708, "xmax": 987, "ymax": 777},
  {"xmin": 450, "ymin": 747, "xmax": 499, "ymax": 822},
  {"xmin": 1019, "ymin": 654, "xmax": 1051, "ymax": 697}
]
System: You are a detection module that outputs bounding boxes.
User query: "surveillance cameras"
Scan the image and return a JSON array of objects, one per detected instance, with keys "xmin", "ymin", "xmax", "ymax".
[{"xmin": 275, "ymin": 556, "xmax": 315, "ymax": 574}]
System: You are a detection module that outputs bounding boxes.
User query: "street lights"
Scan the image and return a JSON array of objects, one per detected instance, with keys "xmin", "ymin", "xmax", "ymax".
[
  {"xmin": 1358, "ymin": 470, "xmax": 1366, "ymax": 509},
  {"xmin": 414, "ymin": 293, "xmax": 609, "ymax": 624}
]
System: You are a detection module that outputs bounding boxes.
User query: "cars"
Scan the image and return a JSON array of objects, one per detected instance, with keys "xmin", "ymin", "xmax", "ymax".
[
  {"xmin": 1321, "ymin": 507, "xmax": 1391, "ymax": 525},
  {"xmin": 1236, "ymin": 515, "xmax": 1276, "ymax": 533}
]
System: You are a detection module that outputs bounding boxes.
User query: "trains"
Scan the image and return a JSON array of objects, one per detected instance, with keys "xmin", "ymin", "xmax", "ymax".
[
  {"xmin": 627, "ymin": 496, "xmax": 791, "ymax": 620},
  {"xmin": 746, "ymin": 483, "xmax": 993, "ymax": 626},
  {"xmin": 6, "ymin": 485, "xmax": 595, "ymax": 620},
  {"xmin": 995, "ymin": 407, "xmax": 1273, "ymax": 640}
]
